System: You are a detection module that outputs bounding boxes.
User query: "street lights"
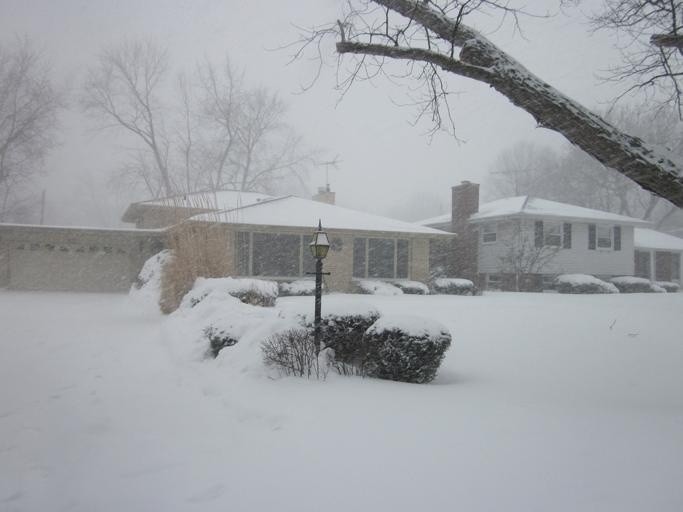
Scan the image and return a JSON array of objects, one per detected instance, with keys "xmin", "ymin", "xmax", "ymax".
[{"xmin": 306, "ymin": 218, "xmax": 331, "ymax": 368}]
